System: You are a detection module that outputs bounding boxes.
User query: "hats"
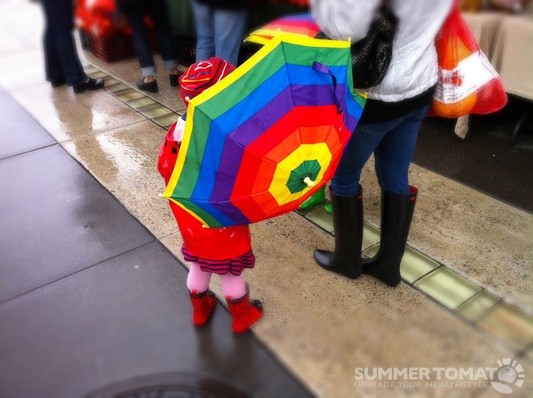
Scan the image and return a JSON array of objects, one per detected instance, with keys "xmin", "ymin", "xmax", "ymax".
[{"xmin": 177, "ymin": 57, "xmax": 235, "ymax": 106}]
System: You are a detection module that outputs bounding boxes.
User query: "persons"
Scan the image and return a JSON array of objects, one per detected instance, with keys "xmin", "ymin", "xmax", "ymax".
[
  {"xmin": 113, "ymin": 0, "xmax": 183, "ymax": 93},
  {"xmin": 157, "ymin": 58, "xmax": 265, "ymax": 333},
  {"xmin": 243, "ymin": 13, "xmax": 321, "ymax": 45},
  {"xmin": 309, "ymin": 0, "xmax": 453, "ymax": 287},
  {"xmin": 28, "ymin": 0, "xmax": 104, "ymax": 94},
  {"xmin": 190, "ymin": 0, "xmax": 250, "ymax": 69}
]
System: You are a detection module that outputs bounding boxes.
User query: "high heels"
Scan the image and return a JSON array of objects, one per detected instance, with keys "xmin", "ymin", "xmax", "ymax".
[{"xmin": 185, "ymin": 287, "xmax": 216, "ymax": 327}]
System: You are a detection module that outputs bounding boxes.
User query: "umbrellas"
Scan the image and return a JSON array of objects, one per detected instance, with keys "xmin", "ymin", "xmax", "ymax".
[{"xmin": 159, "ymin": 28, "xmax": 367, "ymax": 228}]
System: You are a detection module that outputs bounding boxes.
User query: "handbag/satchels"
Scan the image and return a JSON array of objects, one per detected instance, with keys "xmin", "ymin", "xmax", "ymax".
[
  {"xmin": 312, "ymin": 0, "xmax": 400, "ymax": 89},
  {"xmin": 430, "ymin": 0, "xmax": 506, "ymax": 118}
]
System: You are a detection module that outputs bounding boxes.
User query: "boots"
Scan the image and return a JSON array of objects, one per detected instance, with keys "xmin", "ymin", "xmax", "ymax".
[
  {"xmin": 313, "ymin": 184, "xmax": 364, "ymax": 279},
  {"xmin": 360, "ymin": 185, "xmax": 418, "ymax": 287},
  {"xmin": 224, "ymin": 283, "xmax": 263, "ymax": 334}
]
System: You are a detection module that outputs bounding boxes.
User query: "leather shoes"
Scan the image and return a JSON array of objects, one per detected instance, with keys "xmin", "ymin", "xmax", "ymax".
[
  {"xmin": 73, "ymin": 77, "xmax": 104, "ymax": 94},
  {"xmin": 136, "ymin": 76, "xmax": 158, "ymax": 93},
  {"xmin": 50, "ymin": 76, "xmax": 67, "ymax": 87},
  {"xmin": 168, "ymin": 71, "xmax": 184, "ymax": 86}
]
